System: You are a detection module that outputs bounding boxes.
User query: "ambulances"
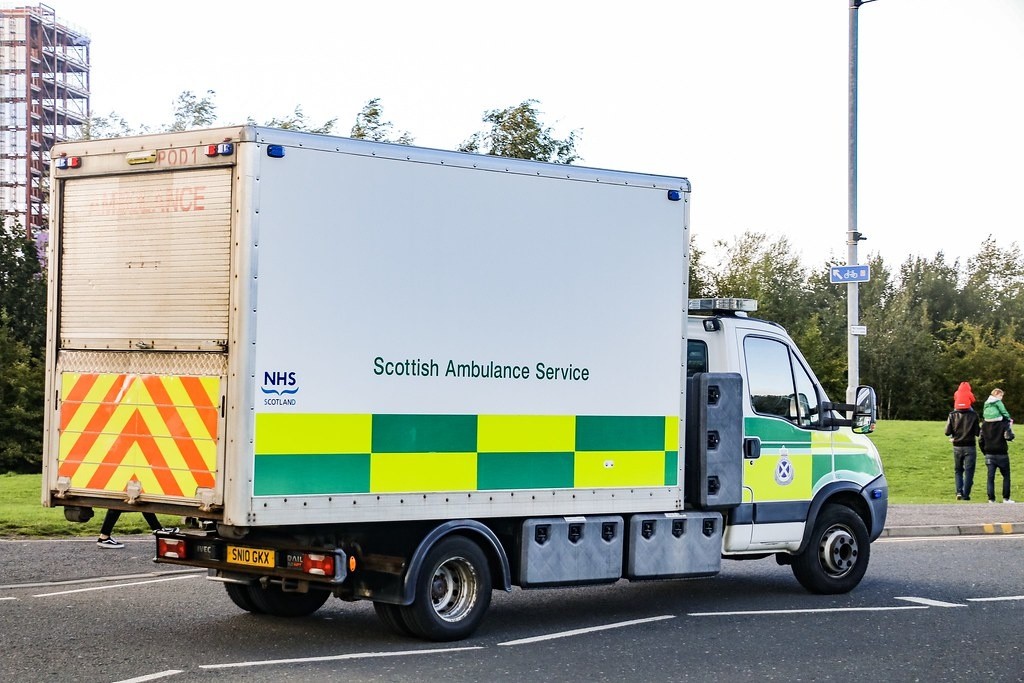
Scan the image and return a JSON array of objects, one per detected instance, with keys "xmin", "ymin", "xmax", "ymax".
[{"xmin": 35, "ymin": 122, "xmax": 888, "ymax": 648}]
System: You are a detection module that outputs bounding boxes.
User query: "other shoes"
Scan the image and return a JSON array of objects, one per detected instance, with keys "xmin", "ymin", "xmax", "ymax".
[
  {"xmin": 1003, "ymin": 499, "xmax": 1015, "ymax": 504},
  {"xmin": 956, "ymin": 492, "xmax": 962, "ymax": 500},
  {"xmin": 963, "ymin": 497, "xmax": 970, "ymax": 500},
  {"xmin": 949, "ymin": 438, "xmax": 954, "ymax": 443},
  {"xmin": 988, "ymin": 500, "xmax": 996, "ymax": 504}
]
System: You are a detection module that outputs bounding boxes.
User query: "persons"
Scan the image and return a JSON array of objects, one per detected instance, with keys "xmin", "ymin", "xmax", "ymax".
[
  {"xmin": 948, "ymin": 382, "xmax": 976, "ymax": 442},
  {"xmin": 983, "ymin": 388, "xmax": 1016, "ymax": 441},
  {"xmin": 96, "ymin": 508, "xmax": 180, "ymax": 549},
  {"xmin": 945, "ymin": 409, "xmax": 980, "ymax": 500},
  {"xmin": 979, "ymin": 417, "xmax": 1016, "ymax": 504}
]
